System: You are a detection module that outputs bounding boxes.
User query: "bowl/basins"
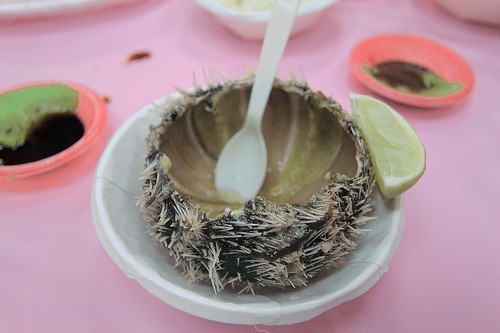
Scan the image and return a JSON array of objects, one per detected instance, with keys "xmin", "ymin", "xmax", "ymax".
[
  {"xmin": 91, "ymin": 87, "xmax": 404, "ymax": 325},
  {"xmin": 198, "ymin": 0, "xmax": 336, "ymax": 42}
]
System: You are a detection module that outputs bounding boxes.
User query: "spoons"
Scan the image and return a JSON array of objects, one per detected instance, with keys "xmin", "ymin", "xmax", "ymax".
[{"xmin": 214, "ymin": 0, "xmax": 301, "ymax": 204}]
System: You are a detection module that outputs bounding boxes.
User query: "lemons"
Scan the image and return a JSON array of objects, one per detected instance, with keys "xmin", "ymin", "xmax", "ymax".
[{"xmin": 351, "ymin": 94, "xmax": 427, "ymax": 198}]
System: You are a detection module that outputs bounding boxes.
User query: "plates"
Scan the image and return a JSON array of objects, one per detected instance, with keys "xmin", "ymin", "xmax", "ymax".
[
  {"xmin": 349, "ymin": 33, "xmax": 477, "ymax": 107},
  {"xmin": 0, "ymin": 80, "xmax": 106, "ymax": 179}
]
